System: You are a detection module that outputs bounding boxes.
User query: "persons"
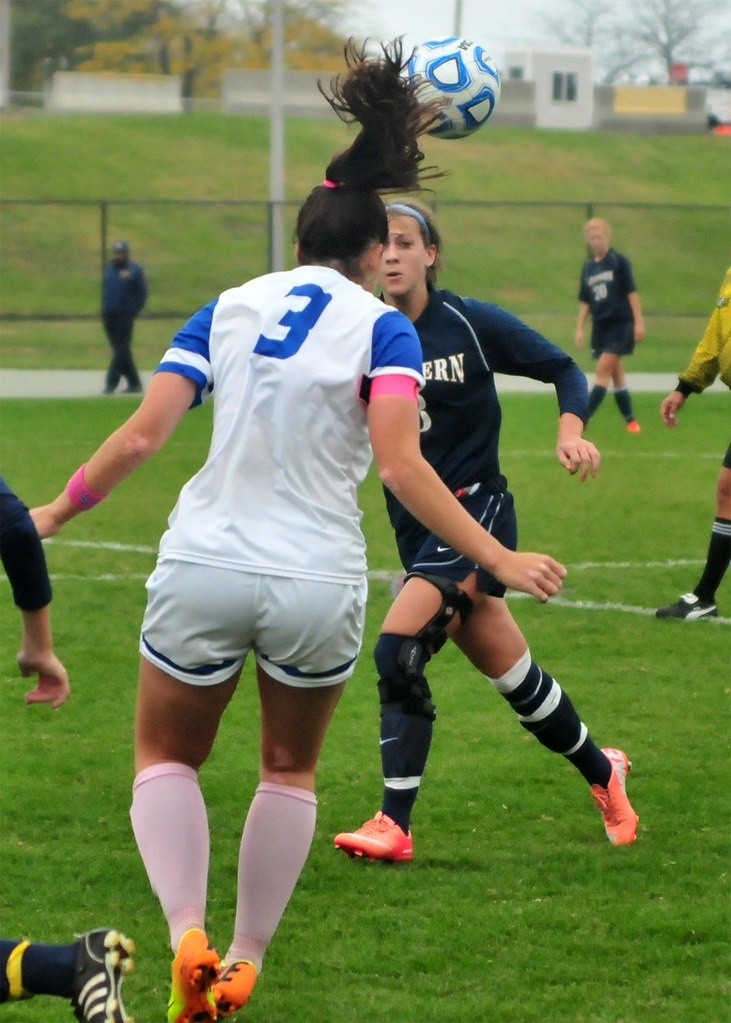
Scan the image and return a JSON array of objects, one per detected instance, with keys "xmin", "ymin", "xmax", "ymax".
[
  {"xmin": 654, "ymin": 266, "xmax": 731, "ymax": 621},
  {"xmin": 0, "ymin": 474, "xmax": 136, "ymax": 1023},
  {"xmin": 28, "ymin": 35, "xmax": 570, "ymax": 1022},
  {"xmin": 333, "ymin": 193, "xmax": 642, "ymax": 861},
  {"xmin": 101, "ymin": 240, "xmax": 149, "ymax": 395},
  {"xmin": 575, "ymin": 217, "xmax": 647, "ymax": 432}
]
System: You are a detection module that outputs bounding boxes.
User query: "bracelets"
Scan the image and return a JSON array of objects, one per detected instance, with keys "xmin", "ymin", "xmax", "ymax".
[{"xmin": 66, "ymin": 464, "xmax": 108, "ymax": 511}]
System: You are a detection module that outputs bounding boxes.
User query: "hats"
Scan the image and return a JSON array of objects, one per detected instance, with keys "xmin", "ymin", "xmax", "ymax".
[{"xmin": 111, "ymin": 241, "xmax": 129, "ymax": 252}]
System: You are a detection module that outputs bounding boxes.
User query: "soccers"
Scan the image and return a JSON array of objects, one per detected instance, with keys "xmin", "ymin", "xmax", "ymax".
[{"xmin": 398, "ymin": 35, "xmax": 502, "ymax": 141}]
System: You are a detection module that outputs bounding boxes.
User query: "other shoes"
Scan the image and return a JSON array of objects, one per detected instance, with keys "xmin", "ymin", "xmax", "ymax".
[
  {"xmin": 103, "ymin": 386, "xmax": 114, "ymax": 395},
  {"xmin": 122, "ymin": 385, "xmax": 142, "ymax": 394},
  {"xmin": 627, "ymin": 420, "xmax": 641, "ymax": 433}
]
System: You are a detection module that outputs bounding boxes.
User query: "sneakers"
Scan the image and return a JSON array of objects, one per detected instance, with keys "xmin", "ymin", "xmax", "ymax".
[
  {"xmin": 656, "ymin": 593, "xmax": 718, "ymax": 621},
  {"xmin": 334, "ymin": 811, "xmax": 414, "ymax": 864},
  {"xmin": 167, "ymin": 929, "xmax": 222, "ymax": 1023},
  {"xmin": 590, "ymin": 747, "xmax": 639, "ymax": 846},
  {"xmin": 71, "ymin": 928, "xmax": 137, "ymax": 1023},
  {"xmin": 213, "ymin": 958, "xmax": 257, "ymax": 1019}
]
